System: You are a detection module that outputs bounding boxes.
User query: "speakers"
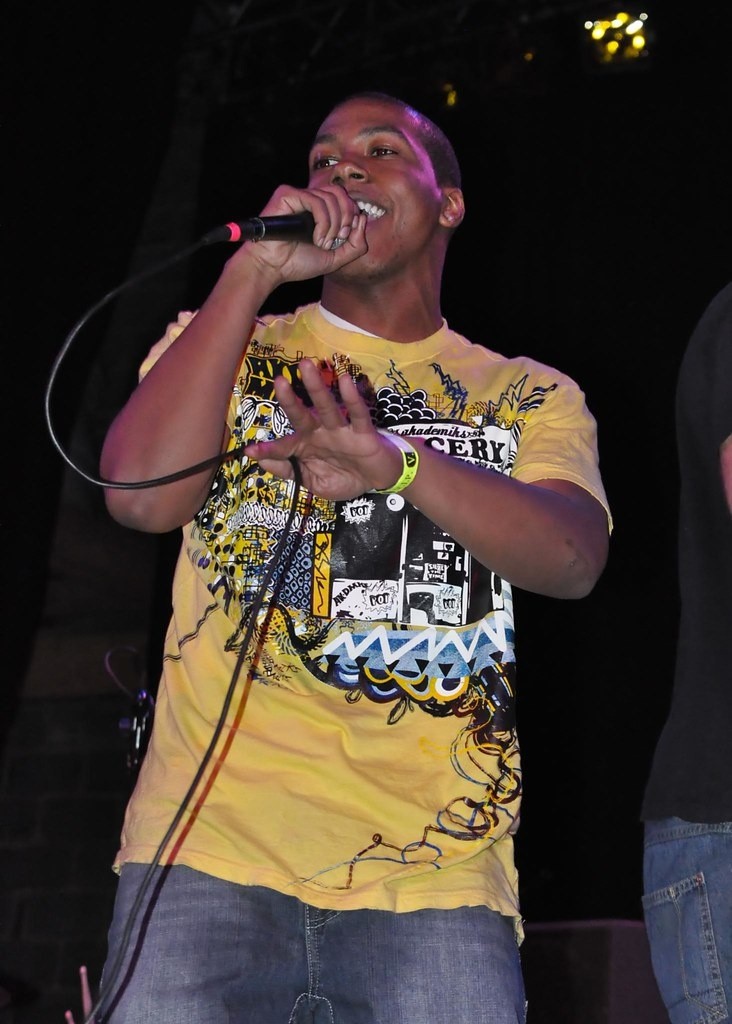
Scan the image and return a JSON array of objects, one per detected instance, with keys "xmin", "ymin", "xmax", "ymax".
[{"xmin": 521, "ymin": 920, "xmax": 672, "ymax": 1024}]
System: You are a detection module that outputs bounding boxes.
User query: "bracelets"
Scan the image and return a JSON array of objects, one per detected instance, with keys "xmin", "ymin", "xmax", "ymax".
[{"xmin": 368, "ymin": 427, "xmax": 419, "ymax": 496}]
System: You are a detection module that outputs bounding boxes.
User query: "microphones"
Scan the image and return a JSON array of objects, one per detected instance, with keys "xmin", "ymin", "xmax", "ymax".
[{"xmin": 213, "ymin": 211, "xmax": 348, "ymax": 249}]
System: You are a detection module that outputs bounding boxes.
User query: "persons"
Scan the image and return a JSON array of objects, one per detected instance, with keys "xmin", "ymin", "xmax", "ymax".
[
  {"xmin": 98, "ymin": 89, "xmax": 613, "ymax": 1024},
  {"xmin": 640, "ymin": 283, "xmax": 732, "ymax": 1024}
]
